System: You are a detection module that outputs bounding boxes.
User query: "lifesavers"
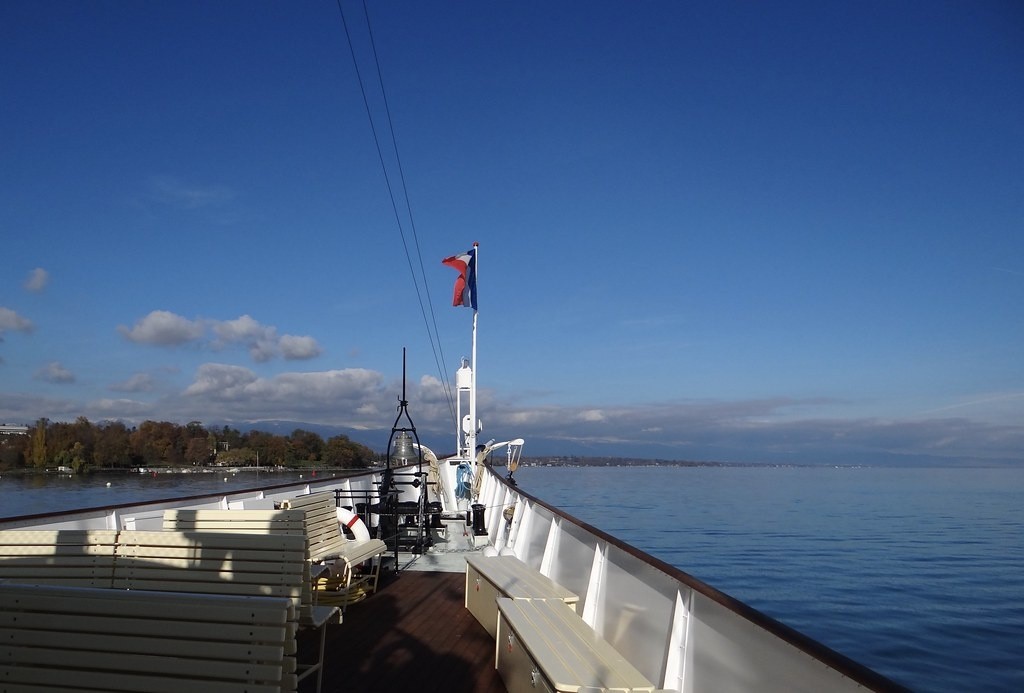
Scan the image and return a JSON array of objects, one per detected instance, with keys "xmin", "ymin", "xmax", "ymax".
[{"xmin": 336, "ymin": 507, "xmax": 370, "ymax": 542}]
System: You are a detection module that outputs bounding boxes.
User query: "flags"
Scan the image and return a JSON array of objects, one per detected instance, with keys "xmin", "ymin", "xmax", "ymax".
[{"xmin": 442, "ymin": 248, "xmax": 478, "ymax": 310}]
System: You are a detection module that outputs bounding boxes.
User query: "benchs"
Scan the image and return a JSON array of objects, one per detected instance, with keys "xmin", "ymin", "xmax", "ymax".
[{"xmin": 0, "ymin": 490, "xmax": 387, "ymax": 692}]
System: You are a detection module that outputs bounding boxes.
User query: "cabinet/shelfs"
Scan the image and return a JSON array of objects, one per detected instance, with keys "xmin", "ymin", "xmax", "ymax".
[
  {"xmin": 464, "ymin": 554, "xmax": 579, "ymax": 640},
  {"xmin": 496, "ymin": 597, "xmax": 655, "ymax": 692}
]
